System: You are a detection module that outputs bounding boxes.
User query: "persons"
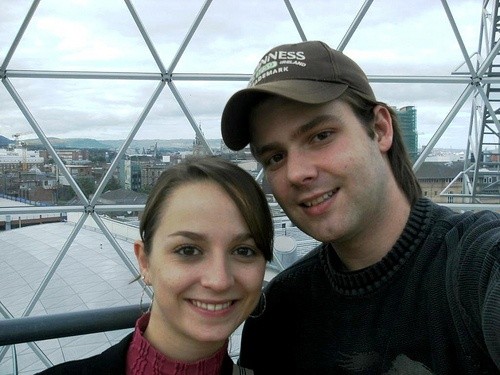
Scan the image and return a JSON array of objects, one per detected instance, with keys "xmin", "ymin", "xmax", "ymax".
[
  {"xmin": 220, "ymin": 41, "xmax": 500, "ymax": 375},
  {"xmin": 27, "ymin": 158, "xmax": 276, "ymax": 375}
]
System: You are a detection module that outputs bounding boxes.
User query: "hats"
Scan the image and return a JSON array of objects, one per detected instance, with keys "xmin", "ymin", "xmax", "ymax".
[{"xmin": 220, "ymin": 41, "xmax": 375, "ymax": 151}]
